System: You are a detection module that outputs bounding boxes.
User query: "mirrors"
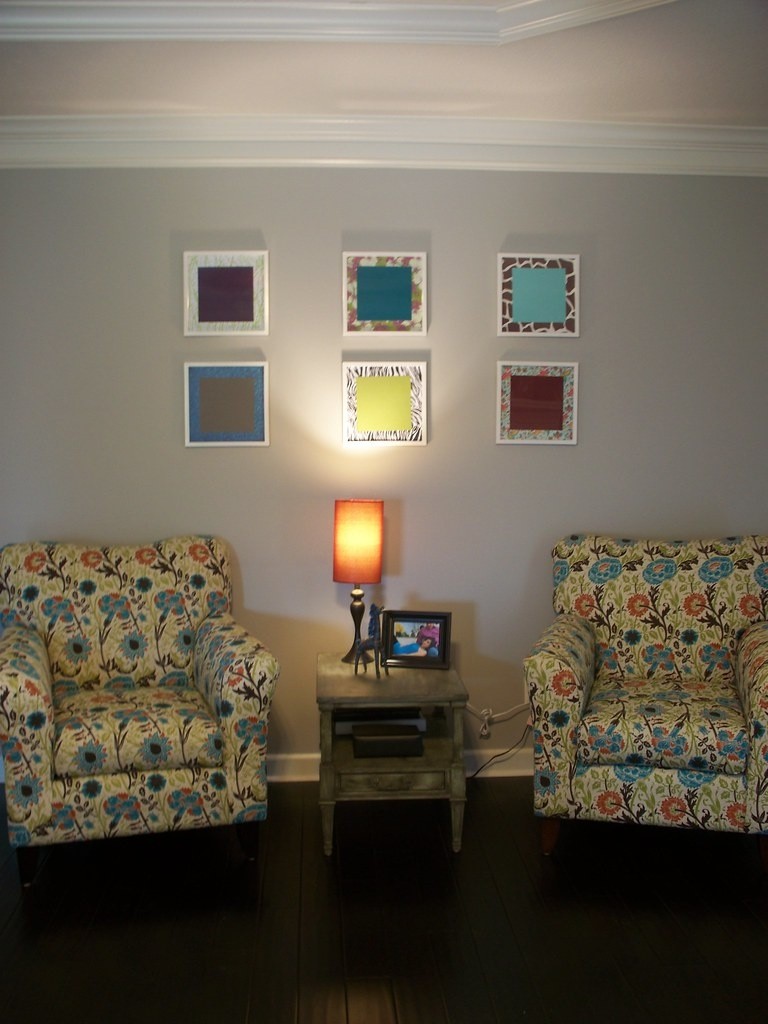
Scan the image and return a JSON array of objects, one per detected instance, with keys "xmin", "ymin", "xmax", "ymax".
[{"xmin": 184, "ymin": 361, "xmax": 269, "ymax": 447}]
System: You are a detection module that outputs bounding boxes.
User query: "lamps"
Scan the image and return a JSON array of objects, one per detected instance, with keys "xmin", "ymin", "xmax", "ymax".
[{"xmin": 333, "ymin": 498, "xmax": 384, "ymax": 664}]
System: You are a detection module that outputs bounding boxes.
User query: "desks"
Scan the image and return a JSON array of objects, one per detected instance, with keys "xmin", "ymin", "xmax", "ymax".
[{"xmin": 317, "ymin": 653, "xmax": 469, "ymax": 855}]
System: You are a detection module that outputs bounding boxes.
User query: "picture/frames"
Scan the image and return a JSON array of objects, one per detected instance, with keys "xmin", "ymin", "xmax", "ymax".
[
  {"xmin": 183, "ymin": 250, "xmax": 270, "ymax": 336},
  {"xmin": 496, "ymin": 361, "xmax": 579, "ymax": 445},
  {"xmin": 342, "ymin": 361, "xmax": 427, "ymax": 446},
  {"xmin": 381, "ymin": 610, "xmax": 452, "ymax": 670},
  {"xmin": 497, "ymin": 253, "xmax": 579, "ymax": 337},
  {"xmin": 341, "ymin": 251, "xmax": 427, "ymax": 336}
]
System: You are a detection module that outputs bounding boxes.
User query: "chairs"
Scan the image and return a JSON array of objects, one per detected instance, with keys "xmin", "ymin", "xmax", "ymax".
[
  {"xmin": 0, "ymin": 534, "xmax": 281, "ymax": 930},
  {"xmin": 523, "ymin": 534, "xmax": 768, "ymax": 856}
]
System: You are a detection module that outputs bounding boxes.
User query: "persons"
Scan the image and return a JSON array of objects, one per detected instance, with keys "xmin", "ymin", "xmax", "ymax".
[{"xmin": 392, "ymin": 622, "xmax": 439, "ymax": 656}]
